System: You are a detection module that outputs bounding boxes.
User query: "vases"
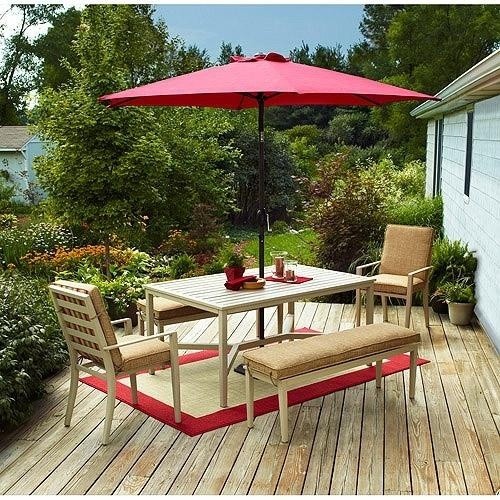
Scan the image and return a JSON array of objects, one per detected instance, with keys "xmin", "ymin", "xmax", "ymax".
[
  {"xmin": 445, "ymin": 299, "xmax": 475, "ymax": 325},
  {"xmin": 224, "ymin": 268, "xmax": 244, "ymax": 280}
]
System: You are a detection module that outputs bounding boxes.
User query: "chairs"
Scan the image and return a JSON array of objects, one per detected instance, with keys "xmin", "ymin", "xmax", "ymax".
[
  {"xmin": 355, "ymin": 225, "xmax": 435, "ymax": 329},
  {"xmin": 47, "ymin": 281, "xmax": 181, "ymax": 446}
]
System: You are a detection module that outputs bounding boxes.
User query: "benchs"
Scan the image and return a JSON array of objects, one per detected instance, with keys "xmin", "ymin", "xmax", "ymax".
[
  {"xmin": 242, "ymin": 322, "xmax": 421, "ymax": 443},
  {"xmin": 137, "ymin": 298, "xmax": 218, "ymax": 369}
]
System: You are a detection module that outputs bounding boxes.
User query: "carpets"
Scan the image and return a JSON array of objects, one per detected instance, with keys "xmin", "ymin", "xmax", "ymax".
[{"xmin": 77, "ymin": 327, "xmax": 431, "ymax": 437}]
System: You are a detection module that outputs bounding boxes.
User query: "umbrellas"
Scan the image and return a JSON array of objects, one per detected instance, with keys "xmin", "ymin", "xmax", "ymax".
[{"xmin": 95, "ymin": 50, "xmax": 444, "ymax": 348}]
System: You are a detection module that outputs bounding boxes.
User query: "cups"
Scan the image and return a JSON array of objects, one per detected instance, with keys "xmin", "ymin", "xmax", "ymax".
[
  {"xmin": 282, "ymin": 258, "xmax": 298, "ymax": 283},
  {"xmin": 269, "ymin": 250, "xmax": 287, "ymax": 280}
]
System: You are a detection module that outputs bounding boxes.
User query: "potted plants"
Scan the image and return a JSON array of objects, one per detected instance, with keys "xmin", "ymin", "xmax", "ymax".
[{"xmin": 431, "ymin": 287, "xmax": 447, "ymax": 314}]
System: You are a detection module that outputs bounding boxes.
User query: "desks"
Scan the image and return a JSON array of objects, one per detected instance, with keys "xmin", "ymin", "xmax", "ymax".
[{"xmin": 142, "ymin": 262, "xmax": 376, "ymax": 409}]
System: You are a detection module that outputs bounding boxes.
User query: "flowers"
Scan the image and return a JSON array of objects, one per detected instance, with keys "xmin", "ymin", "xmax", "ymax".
[
  {"xmin": 430, "ymin": 282, "xmax": 479, "ymax": 306},
  {"xmin": 224, "ymin": 241, "xmax": 258, "ymax": 268}
]
